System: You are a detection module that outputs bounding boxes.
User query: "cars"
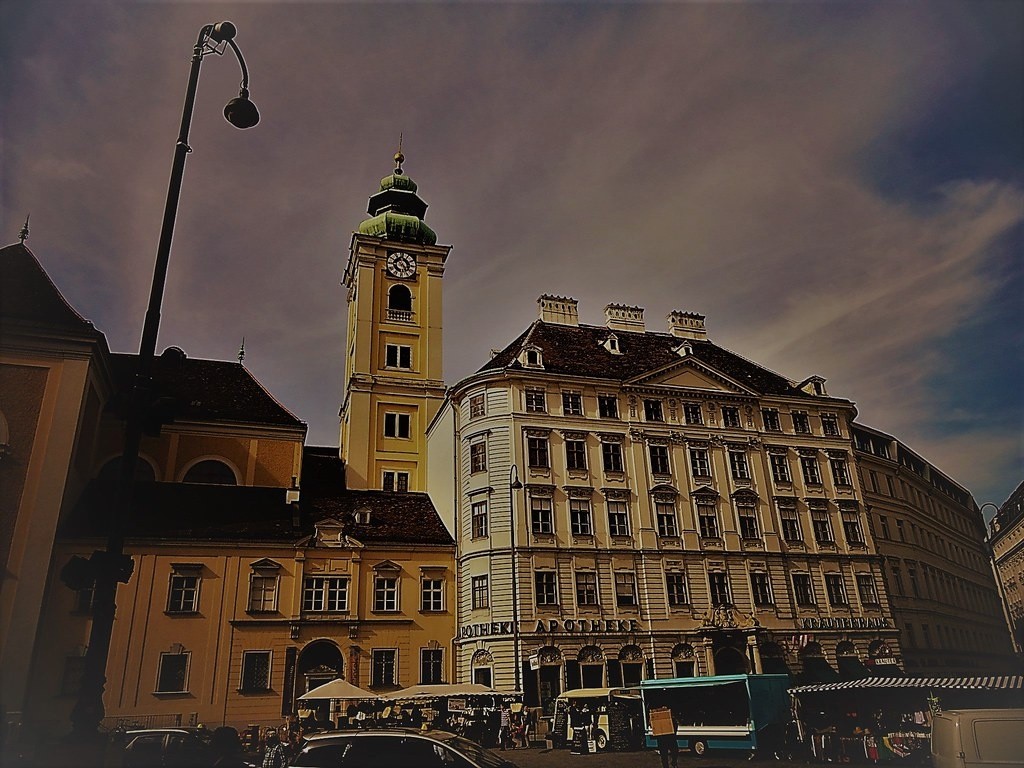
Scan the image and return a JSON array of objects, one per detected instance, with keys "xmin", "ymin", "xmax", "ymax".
[{"xmin": 110, "ymin": 729, "xmax": 256, "ymax": 768}]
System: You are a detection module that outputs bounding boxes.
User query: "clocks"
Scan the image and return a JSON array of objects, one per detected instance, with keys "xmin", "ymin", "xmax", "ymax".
[{"xmin": 388, "ymin": 253, "xmax": 416, "ymax": 279}]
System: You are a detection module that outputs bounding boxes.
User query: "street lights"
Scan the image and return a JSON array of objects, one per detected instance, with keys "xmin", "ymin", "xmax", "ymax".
[
  {"xmin": 980, "ymin": 502, "xmax": 1020, "ymax": 659},
  {"xmin": 65, "ymin": 20, "xmax": 259, "ymax": 768}
]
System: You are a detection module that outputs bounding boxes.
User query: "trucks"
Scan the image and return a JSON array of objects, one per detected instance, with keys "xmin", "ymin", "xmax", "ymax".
[{"xmin": 551, "ymin": 687, "xmax": 642, "ymax": 752}]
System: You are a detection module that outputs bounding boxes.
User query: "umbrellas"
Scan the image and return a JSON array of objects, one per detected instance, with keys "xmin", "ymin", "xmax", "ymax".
[{"xmin": 295, "ymin": 678, "xmax": 380, "ymax": 720}]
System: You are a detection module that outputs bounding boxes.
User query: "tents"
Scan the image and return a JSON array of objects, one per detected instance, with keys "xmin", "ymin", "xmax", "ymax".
[{"xmin": 378, "ymin": 684, "xmax": 524, "ymax": 706}]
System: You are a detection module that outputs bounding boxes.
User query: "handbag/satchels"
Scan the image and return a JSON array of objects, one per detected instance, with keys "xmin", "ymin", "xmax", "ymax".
[{"xmin": 498, "ymin": 729, "xmax": 502, "ymax": 744}]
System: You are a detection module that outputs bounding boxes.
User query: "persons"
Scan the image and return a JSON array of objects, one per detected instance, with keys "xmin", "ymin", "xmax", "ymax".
[
  {"xmin": 401, "ymin": 704, "xmax": 422, "ymax": 728},
  {"xmin": 570, "ymin": 700, "xmax": 595, "ymax": 740},
  {"xmin": 498, "ymin": 704, "xmax": 531, "ymax": 751},
  {"xmin": 261, "ymin": 734, "xmax": 287, "ymax": 768},
  {"xmin": 210, "ymin": 727, "xmax": 243, "ymax": 768},
  {"xmin": 654, "ymin": 705, "xmax": 679, "ymax": 768}
]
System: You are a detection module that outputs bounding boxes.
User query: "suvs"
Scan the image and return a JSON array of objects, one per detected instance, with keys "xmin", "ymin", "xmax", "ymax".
[{"xmin": 289, "ymin": 727, "xmax": 519, "ymax": 768}]
